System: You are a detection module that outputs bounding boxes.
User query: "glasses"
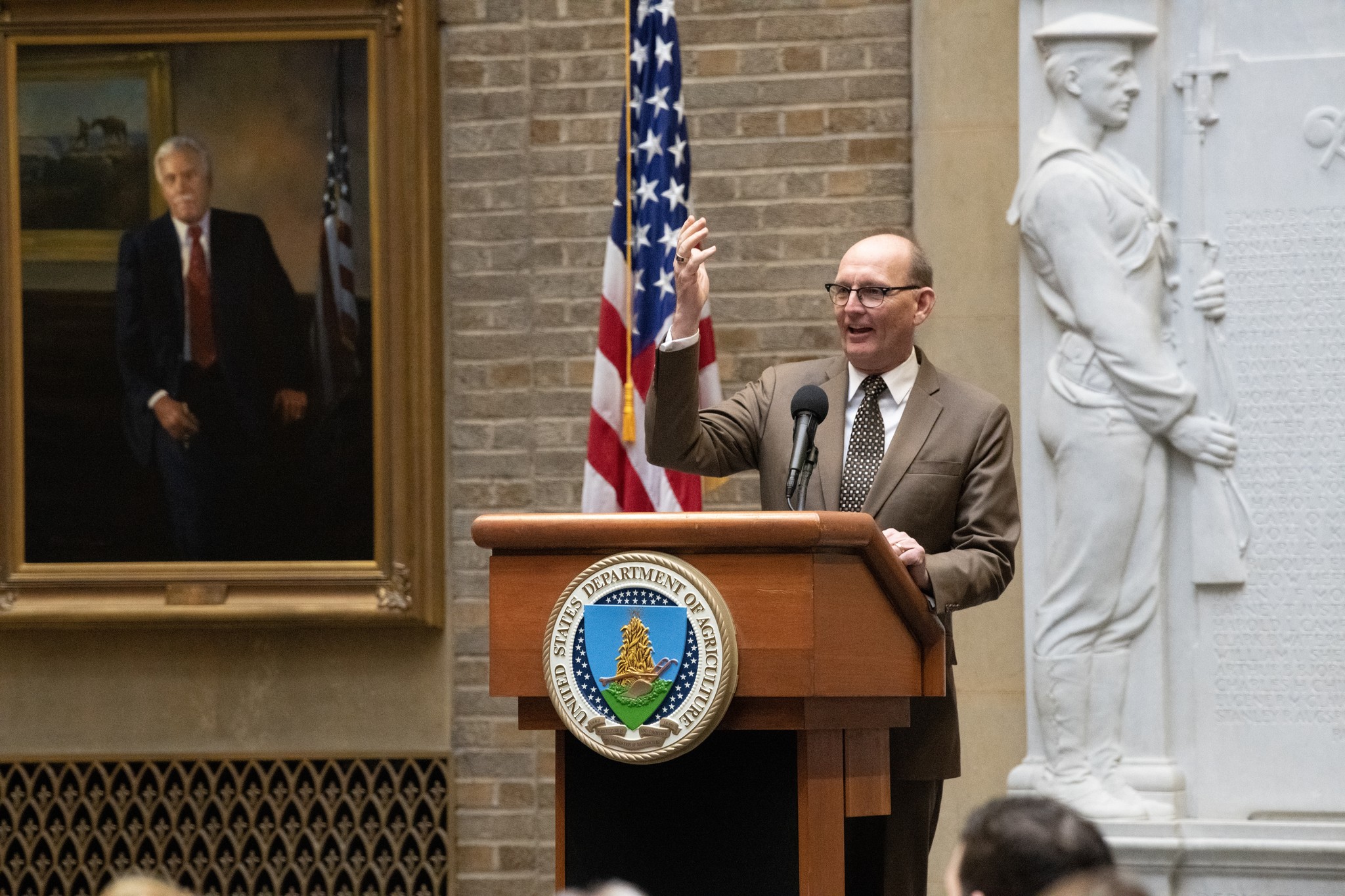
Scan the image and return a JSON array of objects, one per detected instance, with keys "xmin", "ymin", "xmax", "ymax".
[{"xmin": 824, "ymin": 282, "xmax": 922, "ymax": 307}]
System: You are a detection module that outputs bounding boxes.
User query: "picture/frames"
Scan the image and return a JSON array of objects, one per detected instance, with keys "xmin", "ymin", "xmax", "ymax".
[
  {"xmin": 17, "ymin": 52, "xmax": 175, "ymax": 268},
  {"xmin": 0, "ymin": 0, "xmax": 449, "ymax": 638}
]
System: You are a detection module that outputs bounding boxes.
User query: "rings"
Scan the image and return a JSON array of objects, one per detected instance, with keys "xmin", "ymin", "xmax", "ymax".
[
  {"xmin": 675, "ymin": 254, "xmax": 688, "ymax": 263},
  {"xmin": 895, "ymin": 543, "xmax": 905, "ymax": 553}
]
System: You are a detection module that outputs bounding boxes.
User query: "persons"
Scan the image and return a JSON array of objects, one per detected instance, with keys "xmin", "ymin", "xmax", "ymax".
[
  {"xmin": 113, "ymin": 135, "xmax": 311, "ymax": 563},
  {"xmin": 998, "ymin": 13, "xmax": 1248, "ymax": 820},
  {"xmin": 945, "ymin": 795, "xmax": 1113, "ymax": 896},
  {"xmin": 645, "ymin": 217, "xmax": 1021, "ymax": 896}
]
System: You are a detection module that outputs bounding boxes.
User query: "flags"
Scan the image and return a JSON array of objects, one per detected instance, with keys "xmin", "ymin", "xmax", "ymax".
[{"xmin": 583, "ymin": 0, "xmax": 730, "ymax": 516}]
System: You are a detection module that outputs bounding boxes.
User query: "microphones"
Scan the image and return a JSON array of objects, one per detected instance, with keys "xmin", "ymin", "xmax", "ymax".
[{"xmin": 785, "ymin": 385, "xmax": 829, "ymax": 499}]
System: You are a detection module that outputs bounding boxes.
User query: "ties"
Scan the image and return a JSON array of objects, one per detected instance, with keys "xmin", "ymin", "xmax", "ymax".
[
  {"xmin": 187, "ymin": 225, "xmax": 216, "ymax": 370},
  {"xmin": 839, "ymin": 374, "xmax": 887, "ymax": 512}
]
